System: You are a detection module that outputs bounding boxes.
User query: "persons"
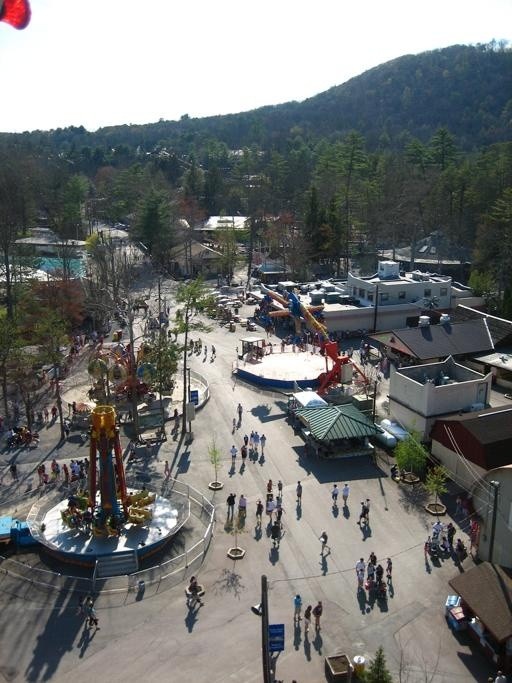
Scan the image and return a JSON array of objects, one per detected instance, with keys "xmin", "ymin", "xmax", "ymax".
[
  {"xmin": 224, "ymin": 478, "xmax": 302, "ymax": 551},
  {"xmin": 366, "ymin": 497, "xmax": 370, "ymax": 511},
  {"xmin": 494, "ymin": 669, "xmax": 507, "ymax": 682},
  {"xmin": 68, "ymin": 327, "xmax": 107, "ymax": 355},
  {"xmin": 293, "ymin": 593, "xmax": 302, "ymax": 622},
  {"xmin": 5, "ymin": 423, "xmax": 40, "ymax": 451},
  {"xmin": 355, "ymin": 551, "xmax": 393, "ymax": 595},
  {"xmin": 317, "ymin": 531, "xmax": 331, "ymax": 555},
  {"xmin": 35, "ymin": 456, "xmax": 90, "ymax": 483},
  {"xmin": 312, "ymin": 600, "xmax": 323, "ymax": 626},
  {"xmin": 163, "ymin": 460, "xmax": 173, "ymax": 476},
  {"xmin": 332, "ymin": 483, "xmax": 339, "ymax": 500},
  {"xmin": 423, "ymin": 494, "xmax": 483, "ymax": 565},
  {"xmin": 356, "ymin": 500, "xmax": 369, "ymax": 524},
  {"xmin": 51, "ymin": 405, "xmax": 57, "ymax": 418},
  {"xmin": 44, "ymin": 406, "xmax": 49, "ymax": 419},
  {"xmin": 189, "ymin": 337, "xmax": 216, "ymax": 360},
  {"xmin": 229, "ymin": 430, "xmax": 266, "ymax": 459},
  {"xmin": 83, "ymin": 596, "xmax": 93, "ymax": 621},
  {"xmin": 189, "ymin": 590, "xmax": 203, "ymax": 605},
  {"xmin": 237, "ymin": 402, "xmax": 242, "ymax": 422},
  {"xmin": 9, "ymin": 461, "xmax": 19, "ymax": 481},
  {"xmin": 342, "ymin": 483, "xmax": 349, "ymax": 503},
  {"xmin": 304, "ymin": 604, "xmax": 311, "ymax": 632},
  {"xmin": 89, "ymin": 605, "xmax": 100, "ymax": 629},
  {"xmin": 174, "ymin": 408, "xmax": 179, "ymax": 421}
]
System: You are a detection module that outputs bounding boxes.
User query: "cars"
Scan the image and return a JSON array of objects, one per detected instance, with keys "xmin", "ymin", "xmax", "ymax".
[{"xmin": 210, "ymin": 290, "xmax": 260, "ymax": 332}]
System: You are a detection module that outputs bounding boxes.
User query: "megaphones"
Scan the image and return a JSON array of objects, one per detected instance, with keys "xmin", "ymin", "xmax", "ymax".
[
  {"xmin": 257, "ymin": 606, "xmax": 263, "ymax": 615},
  {"xmin": 251, "ymin": 603, "xmax": 262, "ymax": 614}
]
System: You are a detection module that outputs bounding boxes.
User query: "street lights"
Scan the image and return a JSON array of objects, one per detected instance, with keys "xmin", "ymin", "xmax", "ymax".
[
  {"xmin": 372, "ymin": 365, "xmax": 379, "ymax": 424},
  {"xmin": 488, "ymin": 479, "xmax": 500, "ymax": 560},
  {"xmin": 156, "ymin": 276, "xmax": 170, "ymax": 412},
  {"xmin": 252, "ymin": 574, "xmax": 272, "ymax": 682},
  {"xmin": 179, "ymin": 289, "xmax": 198, "ymax": 433}
]
